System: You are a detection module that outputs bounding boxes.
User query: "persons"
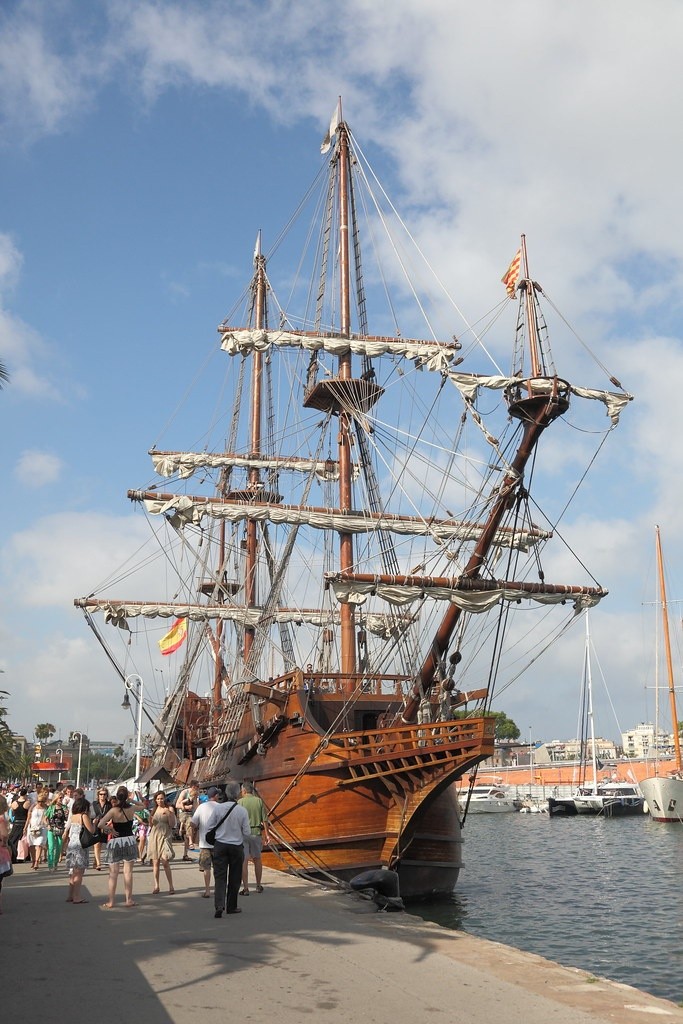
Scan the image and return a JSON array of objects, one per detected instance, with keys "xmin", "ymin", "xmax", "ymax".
[
  {"xmin": 207, "ymin": 782, "xmax": 251, "ymax": 917},
  {"xmin": 0, "ymin": 782, "xmax": 175, "ymax": 870},
  {"xmin": 237, "ymin": 780, "xmax": 271, "ymax": 896},
  {"xmin": 97, "ymin": 786, "xmax": 147, "ymax": 909},
  {"xmin": 0, "ymin": 795, "xmax": 14, "ymax": 916},
  {"xmin": 175, "ymin": 781, "xmax": 209, "ymax": 861},
  {"xmin": 190, "ymin": 787, "xmax": 222, "ymax": 897},
  {"xmin": 65, "ymin": 799, "xmax": 99, "ymax": 904}
]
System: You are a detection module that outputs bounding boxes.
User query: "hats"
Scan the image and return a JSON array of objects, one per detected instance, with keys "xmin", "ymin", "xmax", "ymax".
[
  {"xmin": 208, "ymin": 787, "xmax": 222, "ymax": 797},
  {"xmin": 8, "ymin": 783, "xmax": 22, "ymax": 792}
]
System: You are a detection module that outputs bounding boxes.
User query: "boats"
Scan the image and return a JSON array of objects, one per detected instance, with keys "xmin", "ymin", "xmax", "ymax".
[{"xmin": 457, "ymin": 775, "xmax": 518, "ymax": 813}]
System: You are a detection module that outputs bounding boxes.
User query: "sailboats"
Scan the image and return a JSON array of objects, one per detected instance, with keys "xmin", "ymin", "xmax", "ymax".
[
  {"xmin": 69, "ymin": 90, "xmax": 637, "ymax": 901},
  {"xmin": 520, "ymin": 523, "xmax": 683, "ymax": 824}
]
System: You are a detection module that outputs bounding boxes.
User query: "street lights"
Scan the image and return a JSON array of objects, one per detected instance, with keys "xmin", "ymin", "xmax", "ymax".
[
  {"xmin": 68, "ymin": 731, "xmax": 84, "ymax": 788},
  {"xmin": 120, "ymin": 672, "xmax": 143, "ymax": 794},
  {"xmin": 44, "ymin": 757, "xmax": 53, "ymax": 790},
  {"xmin": 55, "ymin": 748, "xmax": 64, "ymax": 784}
]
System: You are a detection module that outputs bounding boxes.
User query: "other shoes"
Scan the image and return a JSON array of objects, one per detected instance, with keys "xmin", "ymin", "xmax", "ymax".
[
  {"xmin": 256, "ymin": 886, "xmax": 263, "ymax": 893},
  {"xmin": 214, "ymin": 912, "xmax": 222, "ymax": 917},
  {"xmin": 239, "ymin": 889, "xmax": 249, "ymax": 896},
  {"xmin": 227, "ymin": 907, "xmax": 241, "ymax": 913},
  {"xmin": 170, "ymin": 887, "xmax": 174, "ymax": 894},
  {"xmin": 96, "ymin": 865, "xmax": 101, "ymax": 871},
  {"xmin": 153, "ymin": 889, "xmax": 159, "ymax": 894}
]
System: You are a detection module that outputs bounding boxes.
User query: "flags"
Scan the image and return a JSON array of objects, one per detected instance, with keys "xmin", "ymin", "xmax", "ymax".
[
  {"xmin": 158, "ymin": 618, "xmax": 188, "ymax": 656},
  {"xmin": 596, "ymin": 756, "xmax": 604, "ymax": 770}
]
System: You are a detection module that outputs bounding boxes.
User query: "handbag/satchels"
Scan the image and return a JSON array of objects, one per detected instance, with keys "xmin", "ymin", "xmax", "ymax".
[
  {"xmin": 79, "ymin": 813, "xmax": 101, "ymax": 849},
  {"xmin": 16, "ymin": 834, "xmax": 28, "ymax": 859},
  {"xmin": 54, "ymin": 826, "xmax": 64, "ymax": 836},
  {"xmin": 206, "ymin": 831, "xmax": 215, "ymax": 845},
  {"xmin": 164, "ymin": 837, "xmax": 175, "ymax": 862}
]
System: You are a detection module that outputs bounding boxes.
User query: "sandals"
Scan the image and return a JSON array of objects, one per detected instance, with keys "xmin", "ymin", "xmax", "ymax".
[
  {"xmin": 182, "ymin": 855, "xmax": 194, "ymax": 861},
  {"xmin": 189, "ymin": 844, "xmax": 195, "ymax": 850}
]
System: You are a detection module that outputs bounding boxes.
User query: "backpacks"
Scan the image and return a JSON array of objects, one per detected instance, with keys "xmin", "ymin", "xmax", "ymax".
[{"xmin": 11, "ymin": 792, "xmax": 19, "ymax": 801}]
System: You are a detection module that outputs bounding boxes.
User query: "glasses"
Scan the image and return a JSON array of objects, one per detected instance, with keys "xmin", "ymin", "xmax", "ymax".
[
  {"xmin": 100, "ymin": 794, "xmax": 106, "ymax": 795},
  {"xmin": 38, "ymin": 801, "xmax": 44, "ymax": 802},
  {"xmin": 58, "ymin": 797, "xmax": 62, "ymax": 799}
]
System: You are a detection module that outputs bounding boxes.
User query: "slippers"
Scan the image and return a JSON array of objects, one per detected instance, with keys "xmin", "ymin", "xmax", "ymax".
[
  {"xmin": 126, "ymin": 901, "xmax": 139, "ymax": 907},
  {"xmin": 202, "ymin": 893, "xmax": 209, "ymax": 898},
  {"xmin": 103, "ymin": 903, "xmax": 113, "ymax": 908},
  {"xmin": 73, "ymin": 898, "xmax": 89, "ymax": 903},
  {"xmin": 65, "ymin": 900, "xmax": 73, "ymax": 902}
]
